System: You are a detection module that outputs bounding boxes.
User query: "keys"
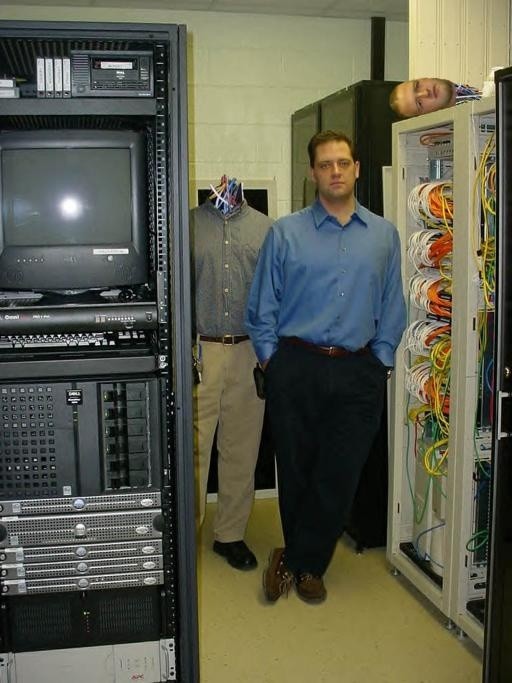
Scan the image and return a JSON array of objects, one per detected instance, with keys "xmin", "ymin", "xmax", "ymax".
[{"xmin": 194, "ymin": 345, "xmax": 203, "ymax": 382}]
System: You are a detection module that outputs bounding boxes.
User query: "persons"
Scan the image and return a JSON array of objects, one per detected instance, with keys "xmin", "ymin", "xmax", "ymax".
[
  {"xmin": 189, "ymin": 186, "xmax": 278, "ymax": 575},
  {"xmin": 241, "ymin": 132, "xmax": 405, "ymax": 606},
  {"xmin": 389, "ymin": 78, "xmax": 459, "ymax": 116}
]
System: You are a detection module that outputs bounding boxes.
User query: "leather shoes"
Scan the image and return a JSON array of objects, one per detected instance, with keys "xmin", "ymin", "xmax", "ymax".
[{"xmin": 213, "ymin": 539, "xmax": 258, "ymax": 571}]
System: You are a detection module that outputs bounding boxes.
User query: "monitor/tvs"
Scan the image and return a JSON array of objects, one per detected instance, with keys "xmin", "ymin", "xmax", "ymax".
[{"xmin": 0, "ymin": 128, "xmax": 148, "ymax": 296}]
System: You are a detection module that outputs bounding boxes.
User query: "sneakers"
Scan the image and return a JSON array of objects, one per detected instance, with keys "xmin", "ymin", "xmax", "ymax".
[
  {"xmin": 296, "ymin": 572, "xmax": 326, "ymax": 603},
  {"xmin": 262, "ymin": 547, "xmax": 294, "ymax": 600}
]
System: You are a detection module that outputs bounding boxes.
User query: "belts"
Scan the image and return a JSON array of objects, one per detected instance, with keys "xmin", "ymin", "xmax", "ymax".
[
  {"xmin": 200, "ymin": 334, "xmax": 251, "ymax": 345},
  {"xmin": 278, "ymin": 335, "xmax": 374, "ymax": 355}
]
